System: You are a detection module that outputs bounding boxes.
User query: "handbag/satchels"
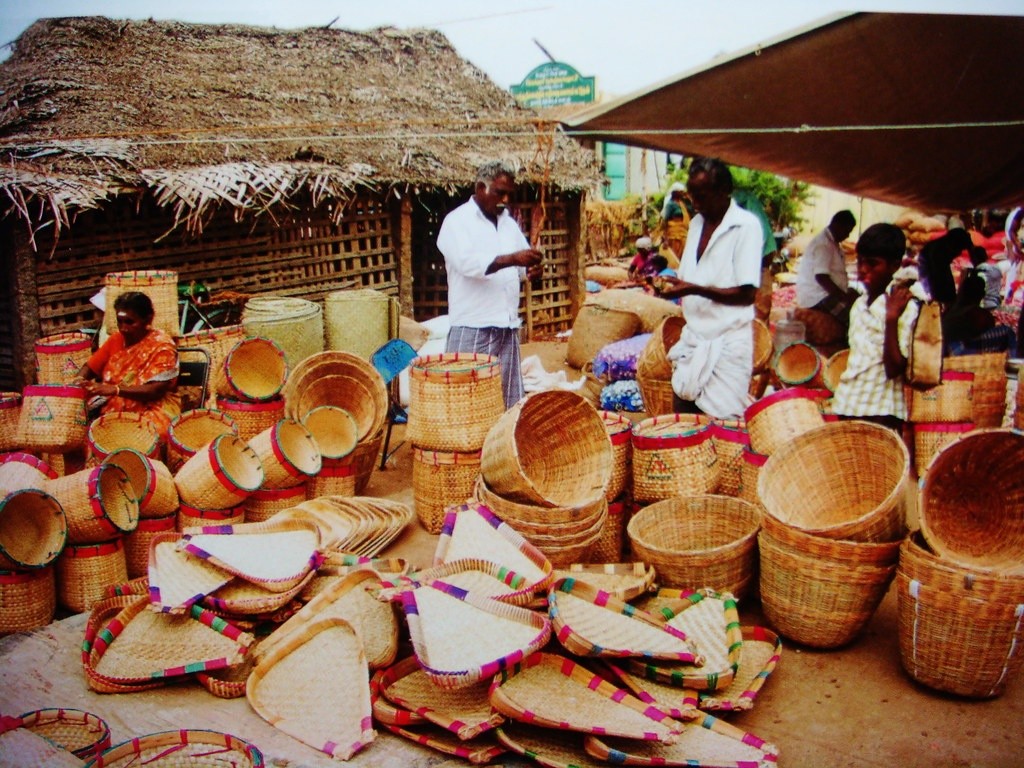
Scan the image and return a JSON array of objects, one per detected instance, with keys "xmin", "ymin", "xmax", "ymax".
[{"xmin": 908, "ymin": 294, "xmax": 943, "ymax": 388}]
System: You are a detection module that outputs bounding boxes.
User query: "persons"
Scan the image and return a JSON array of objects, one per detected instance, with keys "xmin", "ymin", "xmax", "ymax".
[
  {"xmin": 648, "ymin": 255, "xmax": 681, "ymax": 304},
  {"xmin": 830, "ymin": 221, "xmax": 923, "ymax": 438},
  {"xmin": 918, "ymin": 207, "xmax": 1024, "ymax": 372},
  {"xmin": 74, "ymin": 292, "xmax": 181, "ymax": 469},
  {"xmin": 654, "ymin": 156, "xmax": 775, "ymax": 420},
  {"xmin": 794, "ymin": 210, "xmax": 862, "ymax": 326},
  {"xmin": 627, "ymin": 236, "xmax": 656, "ymax": 281},
  {"xmin": 436, "ymin": 161, "xmax": 544, "ymax": 409}
]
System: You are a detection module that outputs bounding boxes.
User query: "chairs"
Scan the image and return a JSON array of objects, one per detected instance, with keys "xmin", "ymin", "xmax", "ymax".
[{"xmin": 178, "ymin": 346, "xmax": 212, "ymax": 410}]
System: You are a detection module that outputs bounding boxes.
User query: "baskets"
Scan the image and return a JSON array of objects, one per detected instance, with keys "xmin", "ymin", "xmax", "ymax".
[
  {"xmin": 378, "ymin": 299, "xmax": 1021, "ymax": 768},
  {"xmin": 1, "ymin": 271, "xmax": 389, "ymax": 768}
]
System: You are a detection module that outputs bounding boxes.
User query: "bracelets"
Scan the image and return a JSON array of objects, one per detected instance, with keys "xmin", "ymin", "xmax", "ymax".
[{"xmin": 115, "ymin": 385, "xmax": 120, "ymax": 395}]
